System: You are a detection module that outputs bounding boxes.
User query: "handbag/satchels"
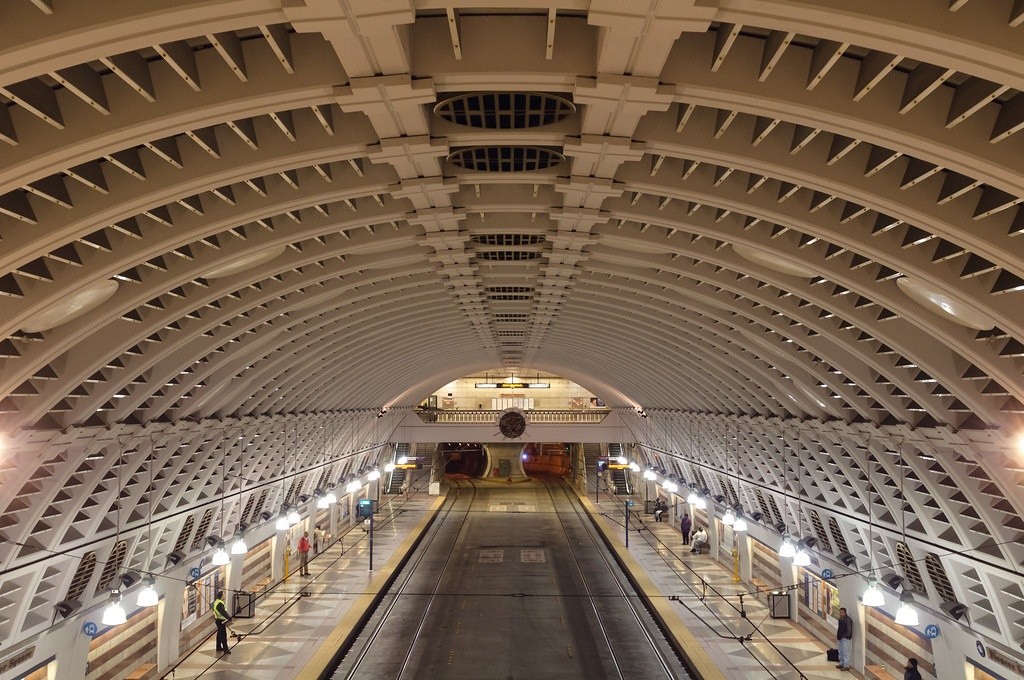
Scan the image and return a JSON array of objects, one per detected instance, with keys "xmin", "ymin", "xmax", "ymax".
[{"xmin": 827, "ymin": 648, "xmax": 840, "ymax": 662}]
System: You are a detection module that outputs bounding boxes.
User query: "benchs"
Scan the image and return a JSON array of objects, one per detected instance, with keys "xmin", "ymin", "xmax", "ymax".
[
  {"xmin": 123, "ymin": 662, "xmax": 158, "ymax": 680},
  {"xmin": 864, "ymin": 663, "xmax": 897, "ymax": 680},
  {"xmin": 689, "ymin": 536, "xmax": 710, "ymax": 553},
  {"xmin": 249, "ymin": 585, "xmax": 266, "ymax": 594},
  {"xmin": 758, "ymin": 587, "xmax": 774, "ymax": 607},
  {"xmin": 322, "ymin": 533, "xmax": 331, "ymax": 539},
  {"xmin": 258, "ymin": 576, "xmax": 274, "ymax": 590},
  {"xmin": 749, "ymin": 577, "xmax": 766, "ymax": 600},
  {"xmin": 660, "ymin": 512, "xmax": 669, "ymax": 518}
]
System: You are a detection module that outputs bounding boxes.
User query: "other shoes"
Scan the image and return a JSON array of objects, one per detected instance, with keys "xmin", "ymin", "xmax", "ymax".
[
  {"xmin": 681, "ymin": 542, "xmax": 688, "ymax": 545},
  {"xmin": 216, "ymin": 647, "xmax": 223, "ymax": 651},
  {"xmin": 305, "ymin": 573, "xmax": 311, "ymax": 575},
  {"xmin": 224, "ymin": 649, "xmax": 231, "ymax": 654},
  {"xmin": 690, "ymin": 548, "xmax": 696, "ymax": 552},
  {"xmin": 836, "ymin": 665, "xmax": 844, "ymax": 669},
  {"xmin": 300, "ymin": 574, "xmax": 304, "ymax": 576},
  {"xmin": 841, "ymin": 667, "xmax": 849, "ymax": 671}
]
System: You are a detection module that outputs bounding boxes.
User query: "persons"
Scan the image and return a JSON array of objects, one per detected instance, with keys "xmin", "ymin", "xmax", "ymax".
[
  {"xmin": 298, "ymin": 531, "xmax": 311, "ymax": 577},
  {"xmin": 904, "ymin": 658, "xmax": 922, "ymax": 680},
  {"xmin": 653, "ymin": 497, "xmax": 667, "ymax": 522},
  {"xmin": 835, "ymin": 607, "xmax": 853, "ymax": 671},
  {"xmin": 680, "ymin": 514, "xmax": 692, "ymax": 545},
  {"xmin": 690, "ymin": 524, "xmax": 707, "ymax": 553},
  {"xmin": 213, "ymin": 590, "xmax": 233, "ymax": 654}
]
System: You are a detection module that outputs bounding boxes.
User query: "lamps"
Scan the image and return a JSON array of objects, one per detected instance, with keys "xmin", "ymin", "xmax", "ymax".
[
  {"xmin": 51, "ymin": 410, "xmax": 408, "ymax": 629},
  {"xmin": 618, "ymin": 410, "xmax": 968, "ymax": 627}
]
